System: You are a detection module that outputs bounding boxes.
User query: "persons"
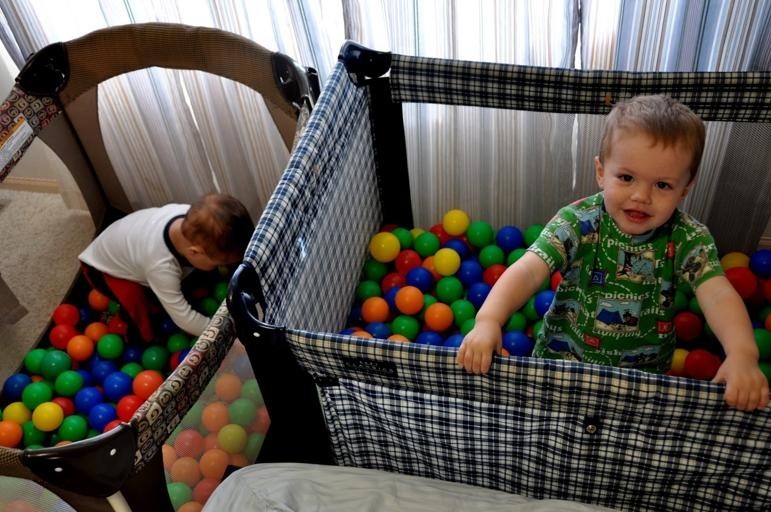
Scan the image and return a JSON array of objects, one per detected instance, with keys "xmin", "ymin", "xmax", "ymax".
[
  {"xmin": 80, "ymin": 192, "xmax": 257, "ymax": 352},
  {"xmin": 456, "ymin": 91, "xmax": 770, "ymax": 413}
]
